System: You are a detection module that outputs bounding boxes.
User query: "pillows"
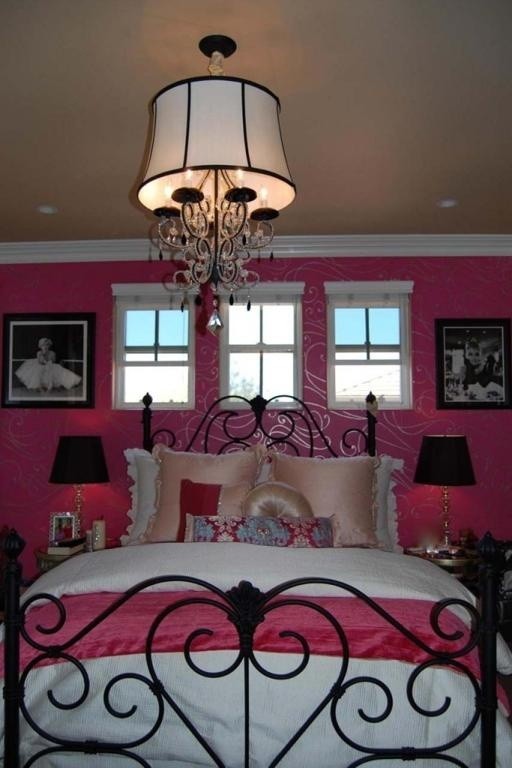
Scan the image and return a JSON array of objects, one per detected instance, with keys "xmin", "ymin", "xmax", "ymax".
[{"xmin": 120, "ymin": 443, "xmax": 404, "ymax": 553}]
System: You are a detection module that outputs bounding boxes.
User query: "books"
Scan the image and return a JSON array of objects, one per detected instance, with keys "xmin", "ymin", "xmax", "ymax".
[{"xmin": 47, "ymin": 543, "xmax": 84, "ymax": 555}]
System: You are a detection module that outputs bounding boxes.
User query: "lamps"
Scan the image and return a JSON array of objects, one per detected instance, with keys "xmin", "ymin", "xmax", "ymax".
[
  {"xmin": 48, "ymin": 435, "xmax": 111, "ymax": 538},
  {"xmin": 412, "ymin": 434, "xmax": 477, "ymax": 555},
  {"xmin": 136, "ymin": 33, "xmax": 297, "ymax": 338}
]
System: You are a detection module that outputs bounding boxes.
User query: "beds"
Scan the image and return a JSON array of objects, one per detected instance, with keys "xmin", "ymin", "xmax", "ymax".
[{"xmin": 0, "ymin": 391, "xmax": 512, "ymax": 768}]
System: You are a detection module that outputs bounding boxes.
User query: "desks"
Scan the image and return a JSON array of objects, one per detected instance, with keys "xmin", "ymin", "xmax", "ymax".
[
  {"xmin": 405, "ymin": 547, "xmax": 480, "ymax": 580},
  {"xmin": 35, "ymin": 546, "xmax": 109, "ymax": 573}
]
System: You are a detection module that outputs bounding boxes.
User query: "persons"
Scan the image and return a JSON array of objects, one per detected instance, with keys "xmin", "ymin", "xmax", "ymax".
[
  {"xmin": 459, "ymin": 339, "xmax": 497, "ymax": 387},
  {"xmin": 64, "ymin": 518, "xmax": 72, "ymax": 536},
  {"xmin": 55, "ymin": 517, "xmax": 65, "ymax": 539},
  {"xmin": 15, "ymin": 337, "xmax": 81, "ymax": 394}
]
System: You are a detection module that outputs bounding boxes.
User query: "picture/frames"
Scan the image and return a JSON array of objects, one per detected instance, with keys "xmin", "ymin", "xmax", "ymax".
[
  {"xmin": 435, "ymin": 317, "xmax": 512, "ymax": 410},
  {"xmin": 1, "ymin": 312, "xmax": 97, "ymax": 409},
  {"xmin": 49, "ymin": 512, "xmax": 80, "ymax": 546}
]
